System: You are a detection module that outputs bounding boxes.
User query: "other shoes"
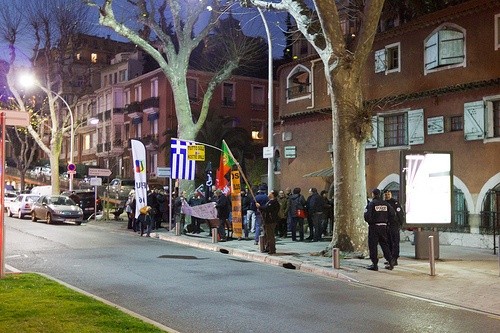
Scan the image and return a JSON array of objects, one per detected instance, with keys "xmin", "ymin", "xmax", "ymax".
[
  {"xmin": 267, "ymin": 252, "xmax": 275, "ymax": 254},
  {"xmin": 263, "ymin": 250, "xmax": 268, "ymax": 252},
  {"xmin": 385, "ymin": 264, "xmax": 392, "ymax": 269},
  {"xmin": 274, "ymin": 232, "xmax": 333, "ymax": 242},
  {"xmin": 384, "ymin": 261, "xmax": 398, "ymax": 265},
  {"xmin": 366, "ymin": 264, "xmax": 378, "ymax": 271},
  {"xmin": 219, "ymin": 238, "xmax": 226, "ymax": 242}
]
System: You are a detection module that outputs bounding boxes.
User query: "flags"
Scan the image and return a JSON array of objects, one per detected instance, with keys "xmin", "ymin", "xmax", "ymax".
[{"xmin": 215, "ymin": 141, "xmax": 236, "ymax": 191}]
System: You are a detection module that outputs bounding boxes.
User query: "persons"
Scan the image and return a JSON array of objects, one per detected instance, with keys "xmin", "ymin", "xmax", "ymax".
[
  {"xmin": 125, "ymin": 185, "xmax": 335, "ymax": 255},
  {"xmin": 363, "ymin": 189, "xmax": 405, "ymax": 272}
]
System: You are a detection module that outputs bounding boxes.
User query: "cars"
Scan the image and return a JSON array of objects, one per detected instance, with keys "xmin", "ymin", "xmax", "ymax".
[
  {"xmin": 30, "ymin": 195, "xmax": 84, "ymax": 226},
  {"xmin": 4, "ymin": 192, "xmax": 18, "ymax": 211},
  {"xmin": 7, "ymin": 193, "xmax": 34, "ymax": 219}
]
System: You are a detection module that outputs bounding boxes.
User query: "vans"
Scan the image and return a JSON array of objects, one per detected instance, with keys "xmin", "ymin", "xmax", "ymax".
[{"xmin": 29, "ymin": 185, "xmax": 53, "ymax": 197}]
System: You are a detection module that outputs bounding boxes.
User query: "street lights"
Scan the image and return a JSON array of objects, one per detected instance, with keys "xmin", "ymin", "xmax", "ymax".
[{"xmin": 19, "ymin": 74, "xmax": 99, "ymax": 191}]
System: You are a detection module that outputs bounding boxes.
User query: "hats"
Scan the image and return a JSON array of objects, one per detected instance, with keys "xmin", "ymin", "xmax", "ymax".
[
  {"xmin": 384, "ymin": 190, "xmax": 390, "ymax": 193},
  {"xmin": 259, "ymin": 186, "xmax": 267, "ymax": 190},
  {"xmin": 216, "ymin": 190, "xmax": 222, "ymax": 193},
  {"xmin": 320, "ymin": 190, "xmax": 328, "ymax": 196},
  {"xmin": 286, "ymin": 187, "xmax": 291, "ymax": 190},
  {"xmin": 371, "ymin": 188, "xmax": 380, "ymax": 195}
]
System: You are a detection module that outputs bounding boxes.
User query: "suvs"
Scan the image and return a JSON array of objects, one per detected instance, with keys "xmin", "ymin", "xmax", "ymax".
[{"xmin": 59, "ymin": 189, "xmax": 102, "ymax": 220}]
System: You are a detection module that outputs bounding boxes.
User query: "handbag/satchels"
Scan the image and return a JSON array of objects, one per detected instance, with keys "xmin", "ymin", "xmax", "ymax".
[
  {"xmin": 140, "ymin": 206, "xmax": 152, "ymax": 215},
  {"xmin": 296, "ymin": 209, "xmax": 307, "ymax": 218}
]
